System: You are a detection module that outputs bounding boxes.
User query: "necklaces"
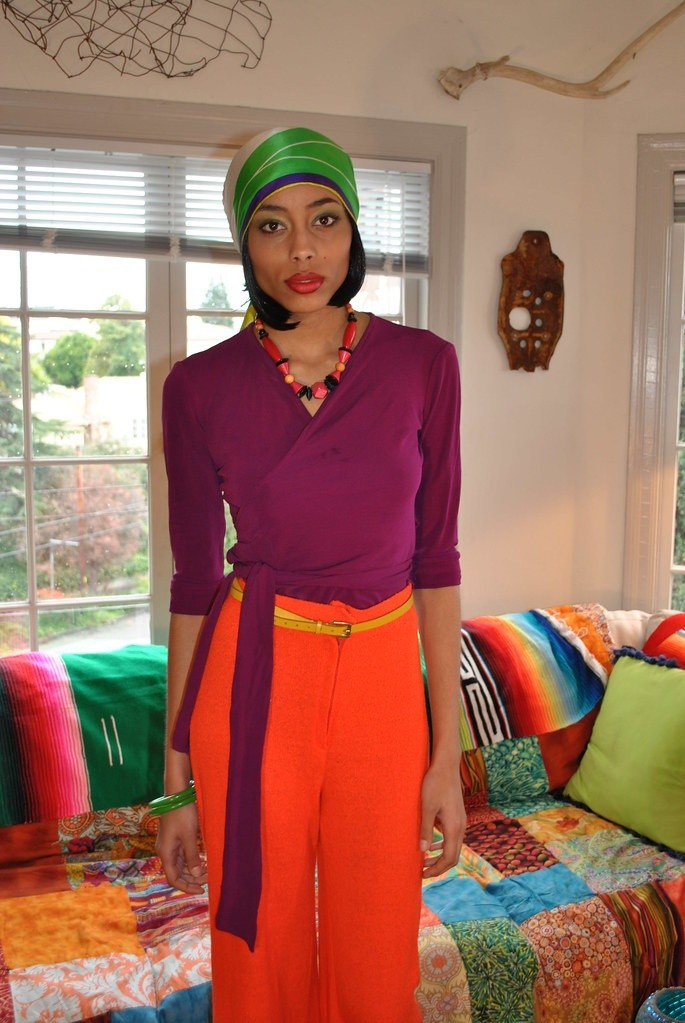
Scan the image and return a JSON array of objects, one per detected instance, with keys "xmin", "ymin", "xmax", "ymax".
[{"xmin": 255, "ymin": 301, "xmax": 357, "ymax": 400}]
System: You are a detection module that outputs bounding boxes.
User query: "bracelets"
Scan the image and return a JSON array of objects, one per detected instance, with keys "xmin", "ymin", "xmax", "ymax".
[{"xmin": 148, "ymin": 781, "xmax": 198, "ymax": 817}]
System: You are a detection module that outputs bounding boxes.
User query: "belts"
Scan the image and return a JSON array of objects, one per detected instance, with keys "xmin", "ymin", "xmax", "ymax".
[{"xmin": 230, "ymin": 578, "xmax": 416, "ymax": 638}]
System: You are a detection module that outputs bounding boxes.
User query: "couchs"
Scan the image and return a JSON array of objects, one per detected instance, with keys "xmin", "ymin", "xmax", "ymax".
[{"xmin": 0, "ymin": 602, "xmax": 685, "ymax": 1023}]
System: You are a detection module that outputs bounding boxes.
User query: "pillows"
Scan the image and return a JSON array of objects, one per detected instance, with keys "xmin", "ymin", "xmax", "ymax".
[{"xmin": 558, "ymin": 645, "xmax": 685, "ymax": 864}]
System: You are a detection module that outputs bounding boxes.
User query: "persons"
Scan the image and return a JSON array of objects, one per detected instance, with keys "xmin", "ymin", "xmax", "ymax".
[{"xmin": 154, "ymin": 125, "xmax": 468, "ymax": 1023}]
